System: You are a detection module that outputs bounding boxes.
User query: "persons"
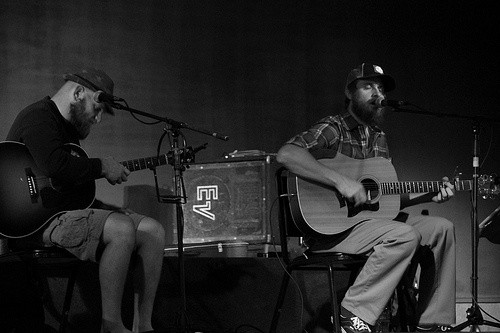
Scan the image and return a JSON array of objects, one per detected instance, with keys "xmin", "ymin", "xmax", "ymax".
[
  {"xmin": 4, "ymin": 67, "xmax": 165, "ymax": 333},
  {"xmin": 274, "ymin": 62, "xmax": 456, "ymax": 333}
]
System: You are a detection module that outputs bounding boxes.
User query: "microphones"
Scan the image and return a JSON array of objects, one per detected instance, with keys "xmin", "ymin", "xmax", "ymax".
[
  {"xmin": 92, "ymin": 91, "xmax": 124, "ymax": 103},
  {"xmin": 373, "ymin": 96, "xmax": 405, "ymax": 107}
]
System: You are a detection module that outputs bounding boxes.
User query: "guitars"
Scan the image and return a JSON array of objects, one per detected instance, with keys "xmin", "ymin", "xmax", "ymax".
[
  {"xmin": 0, "ymin": 142, "xmax": 206, "ymax": 239},
  {"xmin": 286, "ymin": 154, "xmax": 500, "ymax": 234}
]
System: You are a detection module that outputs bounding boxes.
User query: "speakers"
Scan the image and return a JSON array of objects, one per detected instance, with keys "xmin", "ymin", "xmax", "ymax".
[{"xmin": 172, "ymin": 158, "xmax": 285, "ymax": 254}]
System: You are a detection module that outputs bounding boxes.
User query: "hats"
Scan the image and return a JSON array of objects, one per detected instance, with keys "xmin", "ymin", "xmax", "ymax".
[
  {"xmin": 346, "ymin": 62, "xmax": 396, "ymax": 93},
  {"xmin": 64, "ymin": 68, "xmax": 115, "ymax": 117}
]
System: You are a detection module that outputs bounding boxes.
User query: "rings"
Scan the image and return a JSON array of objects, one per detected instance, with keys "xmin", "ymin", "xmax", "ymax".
[{"xmin": 443, "ymin": 197, "xmax": 447, "ymax": 200}]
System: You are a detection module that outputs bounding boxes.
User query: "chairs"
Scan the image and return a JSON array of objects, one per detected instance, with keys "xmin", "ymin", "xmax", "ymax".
[
  {"xmin": 267, "ymin": 166, "xmax": 421, "ymax": 333},
  {"xmin": 0, "ymin": 246, "xmax": 99, "ymax": 333}
]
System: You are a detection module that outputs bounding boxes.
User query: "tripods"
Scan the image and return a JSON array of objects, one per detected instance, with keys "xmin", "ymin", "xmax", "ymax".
[{"xmin": 408, "ymin": 95, "xmax": 500, "ymax": 333}]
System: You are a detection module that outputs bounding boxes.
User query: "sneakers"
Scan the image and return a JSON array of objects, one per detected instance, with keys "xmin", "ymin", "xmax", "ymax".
[
  {"xmin": 415, "ymin": 325, "xmax": 452, "ymax": 333},
  {"xmin": 331, "ymin": 297, "xmax": 374, "ymax": 333}
]
